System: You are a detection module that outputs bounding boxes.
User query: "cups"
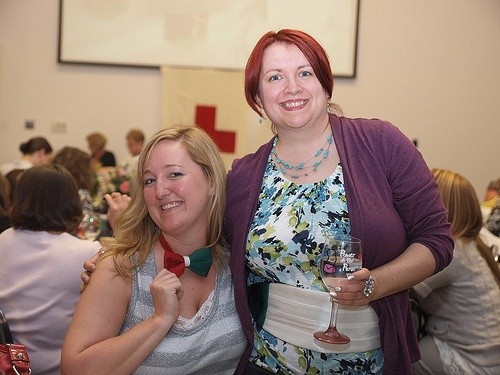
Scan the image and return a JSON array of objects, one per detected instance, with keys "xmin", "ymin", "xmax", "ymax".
[{"xmin": 77, "ymin": 213, "xmax": 102, "ymax": 241}]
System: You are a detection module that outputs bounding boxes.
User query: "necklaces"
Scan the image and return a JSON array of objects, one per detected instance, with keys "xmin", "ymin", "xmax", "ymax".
[{"xmin": 271, "ymin": 133, "xmax": 334, "ymax": 178}]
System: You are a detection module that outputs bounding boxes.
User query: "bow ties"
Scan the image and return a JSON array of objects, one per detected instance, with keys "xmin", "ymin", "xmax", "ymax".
[{"xmin": 159, "ymin": 232, "xmax": 212, "ymax": 279}]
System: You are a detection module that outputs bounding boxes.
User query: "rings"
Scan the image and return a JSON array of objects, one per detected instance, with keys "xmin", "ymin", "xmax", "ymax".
[{"xmin": 364, "ymin": 276, "xmax": 375, "ymax": 298}]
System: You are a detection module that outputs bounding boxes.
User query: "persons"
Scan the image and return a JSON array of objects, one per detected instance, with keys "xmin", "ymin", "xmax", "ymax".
[
  {"xmin": 0, "ymin": 129, "xmax": 145, "ymax": 233},
  {"xmin": 0, "ymin": 164, "xmax": 103, "ymax": 375},
  {"xmin": 412, "ymin": 168, "xmax": 500, "ymax": 375},
  {"xmin": 60, "ymin": 126, "xmax": 248, "ymax": 375},
  {"xmin": 80, "ymin": 28, "xmax": 455, "ymax": 375}
]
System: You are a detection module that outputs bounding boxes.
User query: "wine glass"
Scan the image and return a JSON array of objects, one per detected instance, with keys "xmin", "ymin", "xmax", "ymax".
[{"xmin": 313, "ymin": 234, "xmax": 362, "ymax": 344}]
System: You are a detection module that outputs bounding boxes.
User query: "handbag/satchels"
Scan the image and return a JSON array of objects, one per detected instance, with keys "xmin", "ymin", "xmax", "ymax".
[{"xmin": 0, "ymin": 310, "xmax": 31, "ymax": 375}]
